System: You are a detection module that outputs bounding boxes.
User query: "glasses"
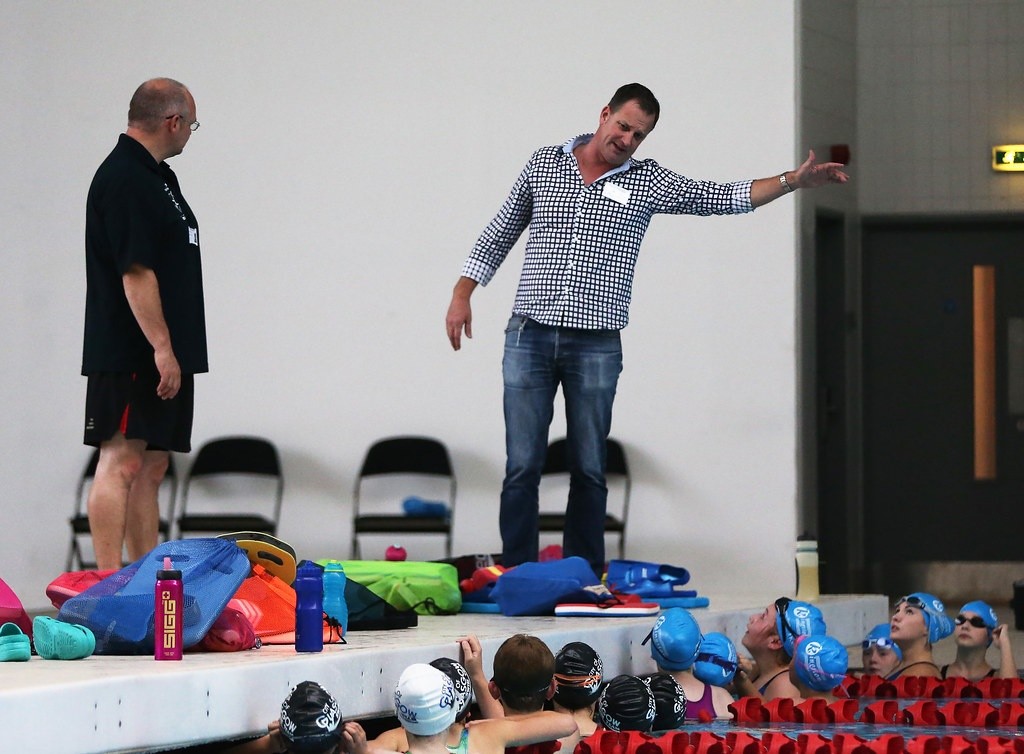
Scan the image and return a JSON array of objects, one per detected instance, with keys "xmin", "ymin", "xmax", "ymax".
[
  {"xmin": 774, "ymin": 597, "xmax": 791, "ymax": 643},
  {"xmin": 861, "ymin": 637, "xmax": 899, "ymax": 652},
  {"xmin": 954, "ymin": 613, "xmax": 987, "ymax": 628},
  {"xmin": 166, "ymin": 113, "xmax": 200, "ymax": 130},
  {"xmin": 794, "ymin": 635, "xmax": 806, "ymax": 656},
  {"xmin": 893, "ymin": 595, "xmax": 932, "ymax": 615}
]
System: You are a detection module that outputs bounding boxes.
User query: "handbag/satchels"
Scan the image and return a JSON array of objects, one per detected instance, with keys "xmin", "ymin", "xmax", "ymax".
[{"xmin": 0, "ymin": 536, "xmax": 695, "ymax": 652}]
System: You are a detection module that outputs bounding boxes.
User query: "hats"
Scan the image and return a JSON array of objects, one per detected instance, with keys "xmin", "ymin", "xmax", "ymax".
[
  {"xmin": 861, "ymin": 623, "xmax": 902, "ymax": 661},
  {"xmin": 647, "ymin": 672, "xmax": 687, "ymax": 732},
  {"xmin": 599, "ymin": 673, "xmax": 656, "ymax": 732},
  {"xmin": 959, "ymin": 601, "xmax": 999, "ymax": 648},
  {"xmin": 394, "ymin": 664, "xmax": 457, "ymax": 735},
  {"xmin": 693, "ymin": 632, "xmax": 738, "ymax": 687},
  {"xmin": 651, "ymin": 607, "xmax": 701, "ymax": 671},
  {"xmin": 906, "ymin": 592, "xmax": 955, "ymax": 644},
  {"xmin": 551, "ymin": 641, "xmax": 603, "ymax": 704},
  {"xmin": 430, "ymin": 657, "xmax": 472, "ymax": 723},
  {"xmin": 279, "ymin": 680, "xmax": 343, "ymax": 744},
  {"xmin": 776, "ymin": 601, "xmax": 826, "ymax": 658},
  {"xmin": 795, "ymin": 634, "xmax": 849, "ymax": 692}
]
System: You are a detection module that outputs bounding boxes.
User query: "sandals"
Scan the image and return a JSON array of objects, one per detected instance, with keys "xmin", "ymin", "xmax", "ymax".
[
  {"xmin": 32, "ymin": 615, "xmax": 96, "ymax": 659},
  {"xmin": 0, "ymin": 622, "xmax": 31, "ymax": 661}
]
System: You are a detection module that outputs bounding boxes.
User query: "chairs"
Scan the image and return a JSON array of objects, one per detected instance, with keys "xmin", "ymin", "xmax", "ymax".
[
  {"xmin": 178, "ymin": 438, "xmax": 284, "ymax": 537},
  {"xmin": 65, "ymin": 448, "xmax": 177, "ymax": 573},
  {"xmin": 537, "ymin": 435, "xmax": 630, "ymax": 560},
  {"xmin": 350, "ymin": 437, "xmax": 457, "ymax": 562}
]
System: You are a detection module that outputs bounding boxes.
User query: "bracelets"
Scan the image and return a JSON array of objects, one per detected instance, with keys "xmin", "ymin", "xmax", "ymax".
[{"xmin": 780, "ymin": 171, "xmax": 793, "ymax": 193}]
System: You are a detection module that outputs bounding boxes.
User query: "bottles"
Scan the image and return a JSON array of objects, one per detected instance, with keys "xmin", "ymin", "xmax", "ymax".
[
  {"xmin": 322, "ymin": 559, "xmax": 348, "ymax": 638},
  {"xmin": 795, "ymin": 529, "xmax": 820, "ymax": 600},
  {"xmin": 385, "ymin": 542, "xmax": 406, "ymax": 561},
  {"xmin": 154, "ymin": 557, "xmax": 184, "ymax": 661},
  {"xmin": 295, "ymin": 559, "xmax": 324, "ymax": 652}
]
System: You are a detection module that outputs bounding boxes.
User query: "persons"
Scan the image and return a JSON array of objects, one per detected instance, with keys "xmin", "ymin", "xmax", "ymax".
[
  {"xmin": 447, "ymin": 84, "xmax": 848, "ymax": 561},
  {"xmin": 884, "ymin": 592, "xmax": 955, "ymax": 681},
  {"xmin": 851, "ymin": 624, "xmax": 903, "ymax": 679},
  {"xmin": 736, "ymin": 597, "xmax": 827, "ymax": 702},
  {"xmin": 456, "ymin": 633, "xmax": 687, "ymax": 754},
  {"xmin": 692, "ymin": 632, "xmax": 737, "ymax": 692},
  {"xmin": 230, "ymin": 681, "xmax": 404, "ymax": 754},
  {"xmin": 395, "ymin": 663, "xmax": 457, "ymax": 754},
  {"xmin": 788, "ymin": 635, "xmax": 848, "ymax": 703},
  {"xmin": 938, "ymin": 601, "xmax": 1018, "ymax": 680},
  {"xmin": 642, "ymin": 607, "xmax": 737, "ymax": 718},
  {"xmin": 81, "ymin": 77, "xmax": 208, "ymax": 569},
  {"xmin": 373, "ymin": 657, "xmax": 577, "ymax": 754}
]
